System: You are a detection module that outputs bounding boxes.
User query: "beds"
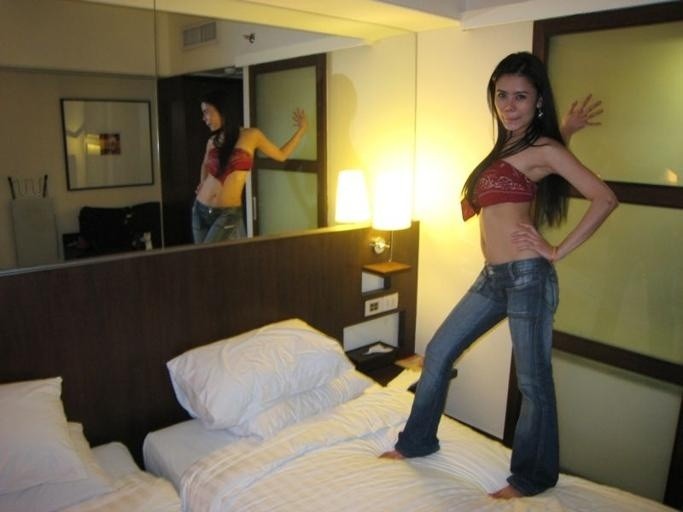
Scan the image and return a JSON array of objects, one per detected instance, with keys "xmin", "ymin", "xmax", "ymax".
[
  {"xmin": 0, "ymin": 443, "xmax": 179, "ymax": 511},
  {"xmin": 143, "ymin": 374, "xmax": 681, "ymax": 511}
]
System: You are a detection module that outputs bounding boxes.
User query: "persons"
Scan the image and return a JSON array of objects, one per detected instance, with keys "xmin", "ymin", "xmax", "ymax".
[
  {"xmin": 190, "ymin": 93, "xmax": 308, "ymax": 246},
  {"xmin": 380, "ymin": 50, "xmax": 620, "ymax": 499}
]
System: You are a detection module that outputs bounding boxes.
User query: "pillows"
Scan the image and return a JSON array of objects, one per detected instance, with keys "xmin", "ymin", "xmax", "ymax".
[
  {"xmin": 0, "ymin": 422, "xmax": 116, "ymax": 511},
  {"xmin": 167, "ymin": 318, "xmax": 375, "ymax": 446},
  {"xmin": 0, "ymin": 376, "xmax": 87, "ymax": 494}
]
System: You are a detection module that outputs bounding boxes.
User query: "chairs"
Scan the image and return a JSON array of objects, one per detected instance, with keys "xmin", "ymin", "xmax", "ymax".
[{"xmin": 59, "ymin": 197, "xmax": 163, "ymax": 265}]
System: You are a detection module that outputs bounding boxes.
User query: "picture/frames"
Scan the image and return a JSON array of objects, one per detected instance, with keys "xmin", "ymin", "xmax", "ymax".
[{"xmin": 58, "ymin": 97, "xmax": 154, "ymax": 191}]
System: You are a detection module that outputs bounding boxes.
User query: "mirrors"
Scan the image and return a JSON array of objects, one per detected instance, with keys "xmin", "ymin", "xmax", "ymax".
[{"xmin": 1, "ymin": 0, "xmax": 418, "ymax": 279}]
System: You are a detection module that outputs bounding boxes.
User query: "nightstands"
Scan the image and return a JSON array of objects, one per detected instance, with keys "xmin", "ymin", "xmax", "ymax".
[{"xmin": 345, "ymin": 340, "xmax": 457, "ymax": 394}]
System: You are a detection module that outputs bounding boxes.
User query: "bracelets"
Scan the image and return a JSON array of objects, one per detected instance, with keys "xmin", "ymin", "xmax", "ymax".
[{"xmin": 550, "ymin": 245, "xmax": 557, "ymax": 264}]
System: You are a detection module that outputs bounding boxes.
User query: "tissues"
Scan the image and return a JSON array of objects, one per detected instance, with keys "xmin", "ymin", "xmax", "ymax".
[{"xmin": 345, "ymin": 341, "xmax": 399, "ymax": 371}]
